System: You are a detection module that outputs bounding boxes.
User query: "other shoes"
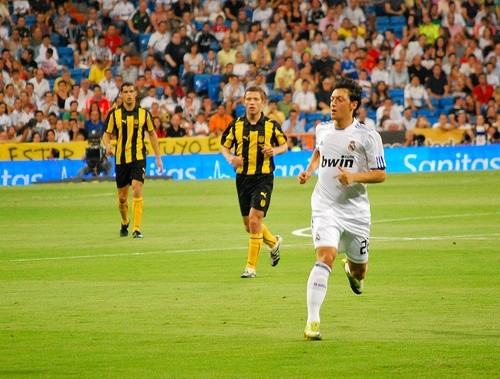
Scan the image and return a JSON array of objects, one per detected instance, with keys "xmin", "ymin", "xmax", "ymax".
[
  {"xmin": 267, "ymin": 235, "xmax": 282, "ymax": 267},
  {"xmin": 341, "ymin": 259, "xmax": 364, "ymax": 295},
  {"xmin": 132, "ymin": 230, "xmax": 143, "ymax": 238},
  {"xmin": 120, "ymin": 218, "xmax": 130, "ymax": 237},
  {"xmin": 303, "ymin": 321, "xmax": 323, "ymax": 341},
  {"xmin": 240, "ymin": 268, "xmax": 257, "ymax": 279}
]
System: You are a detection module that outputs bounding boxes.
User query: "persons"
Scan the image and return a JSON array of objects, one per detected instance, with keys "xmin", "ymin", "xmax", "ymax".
[
  {"xmin": 102, "ymin": 82, "xmax": 163, "ymax": 239},
  {"xmin": 221, "ymin": 86, "xmax": 288, "ymax": 278},
  {"xmin": 297, "ymin": 77, "xmax": 387, "ymax": 340},
  {"xmin": 0, "ymin": 0, "xmax": 500, "ymax": 143}
]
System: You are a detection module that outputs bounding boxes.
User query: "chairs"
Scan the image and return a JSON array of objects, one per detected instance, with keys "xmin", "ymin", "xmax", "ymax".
[
  {"xmin": 8, "ymin": 13, "xmax": 118, "ymax": 90},
  {"xmin": 126, "ymin": 2, "xmax": 477, "ymax": 125}
]
